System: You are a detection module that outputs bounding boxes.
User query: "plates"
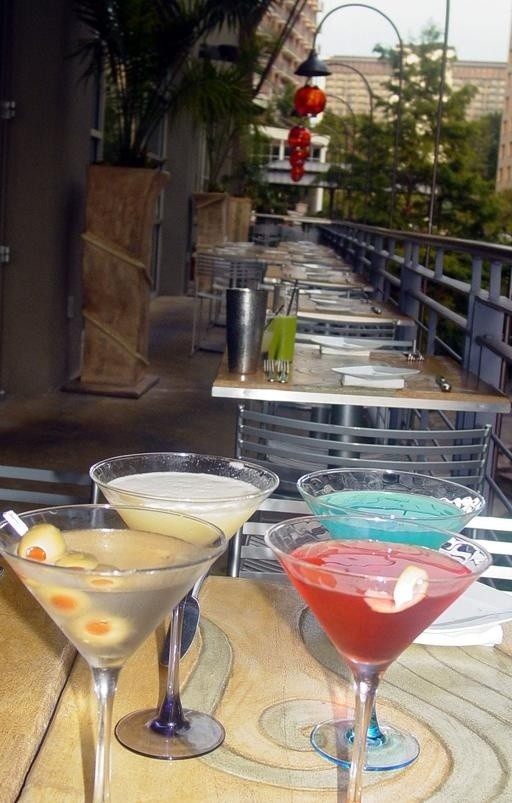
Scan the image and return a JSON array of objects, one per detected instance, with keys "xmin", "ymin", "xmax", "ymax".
[
  {"xmin": 308, "ymin": 296, "xmax": 363, "ymax": 308},
  {"xmin": 425, "ymin": 580, "xmax": 512, "ymax": 633},
  {"xmin": 305, "ymin": 287, "xmax": 348, "ymax": 296},
  {"xmin": 301, "ymin": 262, "xmax": 334, "ymax": 269},
  {"xmin": 330, "ymin": 364, "xmax": 423, "ymax": 382},
  {"xmin": 310, "ymin": 334, "xmax": 386, "ymax": 352}
]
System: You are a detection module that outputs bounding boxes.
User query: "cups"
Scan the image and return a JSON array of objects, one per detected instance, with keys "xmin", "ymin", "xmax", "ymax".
[
  {"xmin": 224, "ymin": 285, "xmax": 268, "ymax": 374},
  {"xmin": 273, "ymin": 282, "xmax": 301, "ymax": 364},
  {"xmin": 263, "ymin": 313, "xmax": 278, "ymax": 361}
]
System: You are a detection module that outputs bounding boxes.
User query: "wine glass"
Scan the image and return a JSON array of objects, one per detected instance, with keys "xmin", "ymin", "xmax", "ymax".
[
  {"xmin": 83, "ymin": 450, "xmax": 282, "ymax": 761},
  {"xmin": 260, "ymin": 516, "xmax": 493, "ymax": 802},
  {"xmin": 293, "ymin": 463, "xmax": 486, "ymax": 778},
  {"xmin": 0, "ymin": 505, "xmax": 227, "ymax": 803}
]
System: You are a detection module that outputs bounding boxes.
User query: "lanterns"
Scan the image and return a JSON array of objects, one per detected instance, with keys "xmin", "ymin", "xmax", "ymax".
[
  {"xmin": 294, "ymin": 82, "xmax": 326, "ymax": 115},
  {"xmin": 287, "ymin": 124, "xmax": 311, "ymax": 181}
]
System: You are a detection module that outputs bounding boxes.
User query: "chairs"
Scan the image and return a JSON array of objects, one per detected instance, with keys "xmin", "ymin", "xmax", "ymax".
[
  {"xmin": 0, "ymin": 458, "xmax": 105, "ymax": 546},
  {"xmin": 226, "ymin": 491, "xmax": 511, "ymax": 604}
]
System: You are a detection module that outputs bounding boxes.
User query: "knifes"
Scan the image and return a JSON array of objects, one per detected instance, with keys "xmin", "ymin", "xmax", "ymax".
[{"xmin": 158, "ymin": 571, "xmax": 206, "ymax": 668}]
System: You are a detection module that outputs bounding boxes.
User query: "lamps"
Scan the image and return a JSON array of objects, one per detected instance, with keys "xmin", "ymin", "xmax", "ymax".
[{"xmin": 287, "ymin": 0, "xmax": 409, "ymax": 227}]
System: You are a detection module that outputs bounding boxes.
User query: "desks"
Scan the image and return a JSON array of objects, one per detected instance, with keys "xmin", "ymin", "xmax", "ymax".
[
  {"xmin": 20, "ymin": 573, "xmax": 511, "ymax": 803},
  {"xmin": 0, "ymin": 552, "xmax": 78, "ymax": 803}
]
